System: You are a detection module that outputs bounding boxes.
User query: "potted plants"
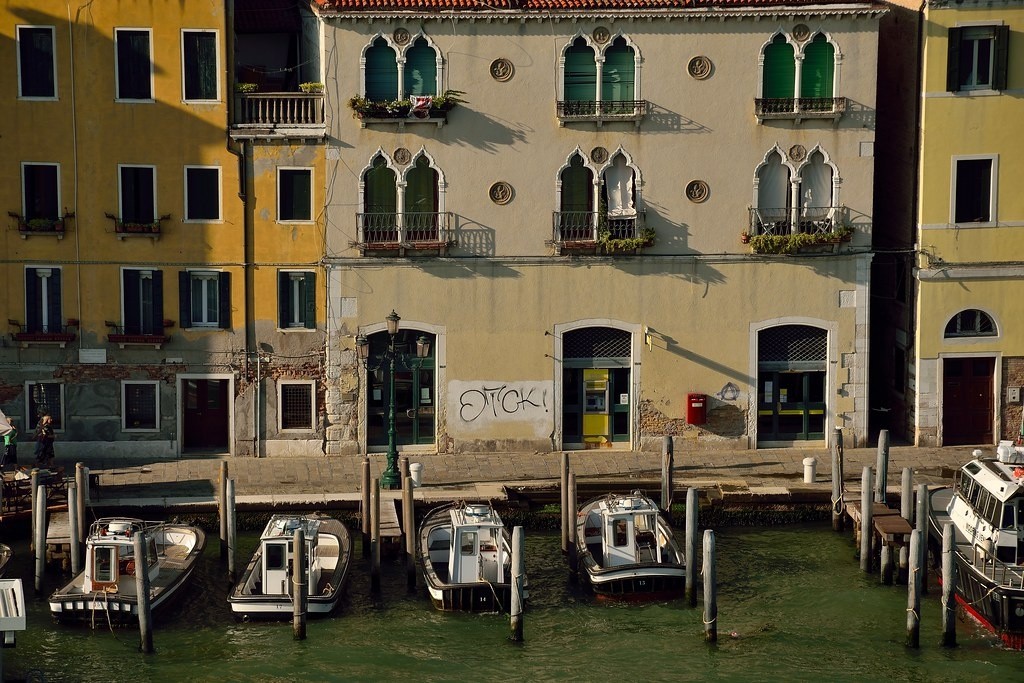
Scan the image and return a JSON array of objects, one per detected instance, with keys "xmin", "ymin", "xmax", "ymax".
[
  {"xmin": 19, "ymin": 219, "xmax": 64, "ymax": 232},
  {"xmin": 114, "ymin": 222, "xmax": 160, "ymax": 233}
]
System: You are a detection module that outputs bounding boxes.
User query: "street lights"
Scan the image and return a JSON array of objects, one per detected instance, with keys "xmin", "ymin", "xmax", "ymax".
[{"xmin": 356, "ymin": 309, "xmax": 430, "ymax": 490}]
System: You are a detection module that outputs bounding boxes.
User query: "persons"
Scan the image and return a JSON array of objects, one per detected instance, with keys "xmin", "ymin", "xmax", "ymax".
[
  {"xmin": 0, "ymin": 417, "xmax": 18, "ymax": 468},
  {"xmin": 34, "ymin": 414, "xmax": 55, "ymax": 469},
  {"xmin": 980, "ymin": 537, "xmax": 994, "ymax": 566}
]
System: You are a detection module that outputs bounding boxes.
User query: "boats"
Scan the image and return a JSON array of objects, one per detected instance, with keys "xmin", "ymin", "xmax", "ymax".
[
  {"xmin": 417, "ymin": 497, "xmax": 529, "ymax": 614},
  {"xmin": 925, "ymin": 440, "xmax": 1024, "ymax": 653},
  {"xmin": 576, "ymin": 488, "xmax": 686, "ymax": 601},
  {"xmin": 48, "ymin": 516, "xmax": 207, "ymax": 629},
  {"xmin": 226, "ymin": 511, "xmax": 354, "ymax": 622}
]
